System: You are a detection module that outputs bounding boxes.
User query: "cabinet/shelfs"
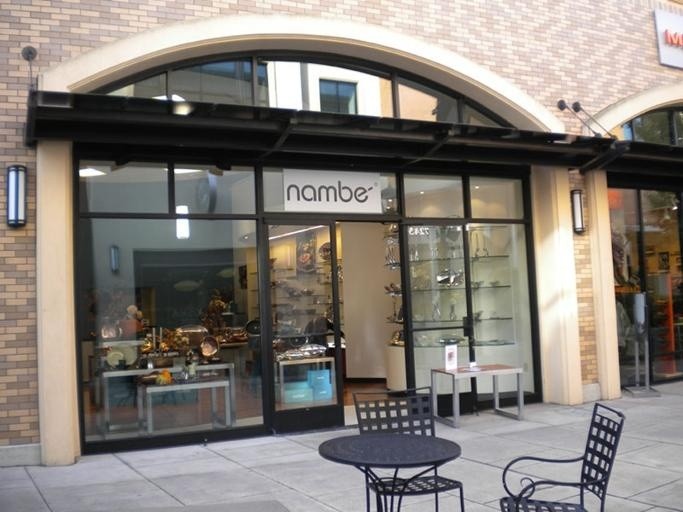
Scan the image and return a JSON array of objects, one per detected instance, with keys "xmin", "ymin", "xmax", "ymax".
[
  {"xmin": 379, "ymin": 224, "xmax": 516, "ymax": 396},
  {"xmin": 248, "ymin": 261, "xmax": 341, "ymax": 342}
]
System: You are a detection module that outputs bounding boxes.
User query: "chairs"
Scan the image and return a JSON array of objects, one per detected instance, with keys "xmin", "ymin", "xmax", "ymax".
[
  {"xmin": 497, "ymin": 402, "xmax": 624, "ymax": 512},
  {"xmin": 354, "ymin": 384, "xmax": 466, "ymax": 511}
]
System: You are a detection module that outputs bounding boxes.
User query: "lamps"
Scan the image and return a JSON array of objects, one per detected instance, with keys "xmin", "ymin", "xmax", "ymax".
[
  {"xmin": 22, "ymin": 46, "xmax": 36, "ymax": 91},
  {"xmin": 555, "ymin": 100, "xmax": 618, "ymax": 141},
  {"xmin": 108, "ymin": 245, "xmax": 121, "ymax": 277},
  {"xmin": 569, "ymin": 187, "xmax": 586, "ymax": 233},
  {"xmin": 4, "ymin": 164, "xmax": 28, "ymax": 228},
  {"xmin": 379, "ymin": 177, "xmax": 396, "ymax": 212}
]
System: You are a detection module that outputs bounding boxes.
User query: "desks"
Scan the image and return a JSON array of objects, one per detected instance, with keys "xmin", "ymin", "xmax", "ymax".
[
  {"xmin": 318, "ymin": 431, "xmax": 461, "ymax": 511},
  {"xmin": 82, "ymin": 331, "xmax": 249, "ymax": 430},
  {"xmin": 430, "ymin": 364, "xmax": 528, "ymax": 428},
  {"xmin": 270, "ymin": 357, "xmax": 336, "ymax": 405}
]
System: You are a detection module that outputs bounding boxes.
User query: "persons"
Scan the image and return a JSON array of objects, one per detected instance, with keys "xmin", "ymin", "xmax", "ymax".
[
  {"xmin": 304, "ymin": 317, "xmax": 346, "ymax": 369},
  {"xmin": 118, "ymin": 305, "xmax": 143, "ymax": 336}
]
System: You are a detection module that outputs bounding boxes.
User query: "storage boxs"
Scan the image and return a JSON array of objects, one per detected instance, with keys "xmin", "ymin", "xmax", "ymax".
[{"xmin": 281, "ymin": 367, "xmax": 332, "ymax": 404}]
